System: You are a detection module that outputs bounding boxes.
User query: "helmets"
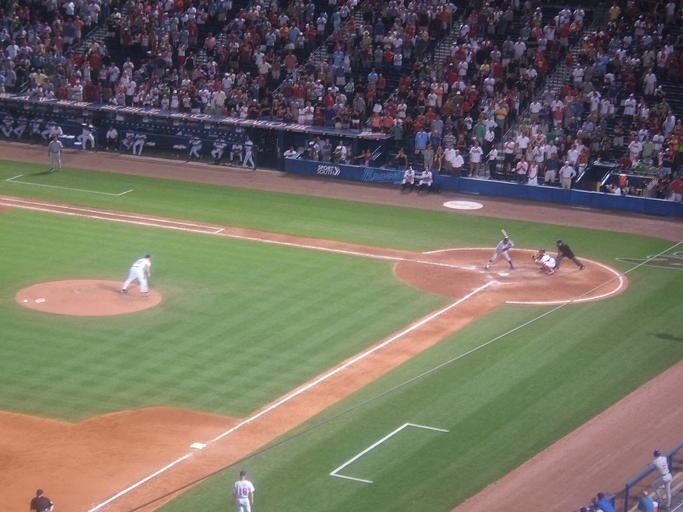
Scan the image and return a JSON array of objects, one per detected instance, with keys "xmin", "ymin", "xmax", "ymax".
[
  {"xmin": 504, "ymin": 236, "xmax": 562, "ymax": 253},
  {"xmin": 580, "ymin": 449, "xmax": 662, "ymax": 512}
]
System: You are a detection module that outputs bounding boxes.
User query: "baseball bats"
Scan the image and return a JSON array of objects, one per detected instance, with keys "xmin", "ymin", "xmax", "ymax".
[{"xmin": 501, "ymin": 229, "xmax": 514, "ymax": 246}]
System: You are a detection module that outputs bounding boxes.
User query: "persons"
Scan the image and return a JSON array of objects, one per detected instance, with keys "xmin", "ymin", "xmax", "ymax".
[
  {"xmin": 591, "ymin": 492, "xmax": 617, "ymax": 512},
  {"xmin": 590, "ymin": 503, "xmax": 603, "ymax": 512},
  {"xmin": 534, "ymin": 248, "xmax": 556, "ymax": 275},
  {"xmin": 2, "ymin": 0, "xmax": 682, "ymax": 197},
  {"xmin": 485, "ymin": 236, "xmax": 514, "ymax": 269},
  {"xmin": 648, "ymin": 448, "xmax": 672, "ymax": 507},
  {"xmin": 637, "ymin": 489, "xmax": 656, "ymax": 512},
  {"xmin": 554, "ymin": 240, "xmax": 583, "ymax": 270},
  {"xmin": 119, "ymin": 252, "xmax": 151, "ymax": 296},
  {"xmin": 231, "ymin": 471, "xmax": 254, "ymax": 512},
  {"xmin": 30, "ymin": 488, "xmax": 54, "ymax": 511}
]
